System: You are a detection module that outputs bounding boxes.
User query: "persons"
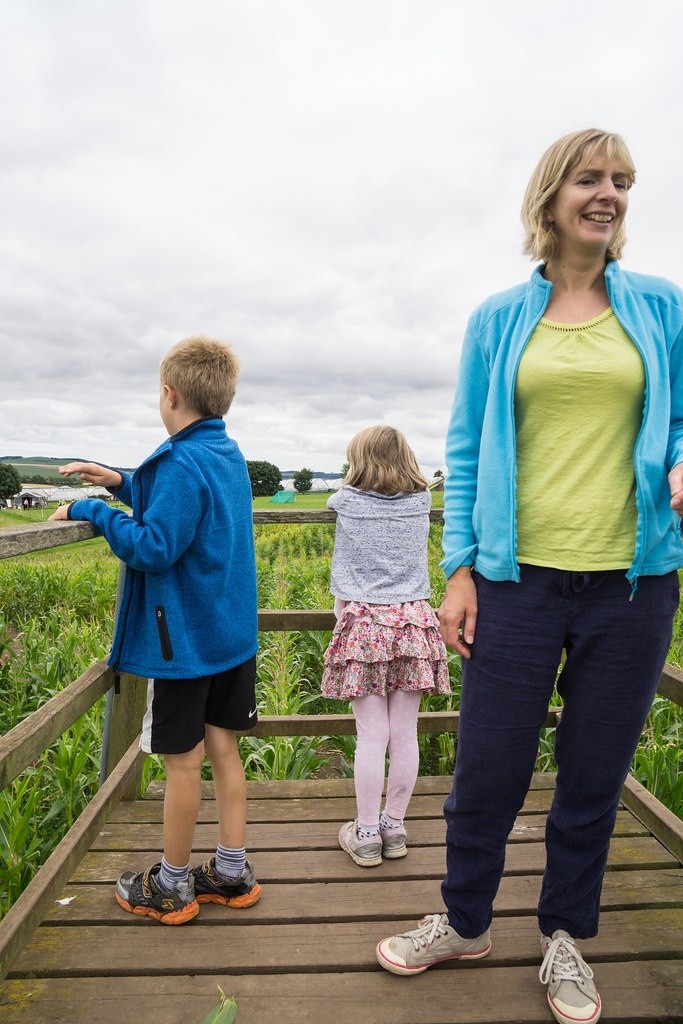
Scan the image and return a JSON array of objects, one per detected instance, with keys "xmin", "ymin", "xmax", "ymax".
[
  {"xmin": 372, "ymin": 130, "xmax": 683, "ymax": 1024},
  {"xmin": 49, "ymin": 338, "xmax": 259, "ymax": 924},
  {"xmin": 324, "ymin": 426, "xmax": 449, "ymax": 867}
]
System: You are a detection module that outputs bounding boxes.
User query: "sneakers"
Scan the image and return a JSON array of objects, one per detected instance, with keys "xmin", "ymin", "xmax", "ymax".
[
  {"xmin": 339, "ymin": 818, "xmax": 384, "ymax": 866},
  {"xmin": 115, "ymin": 863, "xmax": 199, "ymax": 925},
  {"xmin": 538, "ymin": 930, "xmax": 602, "ymax": 1024},
  {"xmin": 378, "ymin": 812, "xmax": 408, "ymax": 858},
  {"xmin": 376, "ymin": 912, "xmax": 492, "ymax": 975},
  {"xmin": 188, "ymin": 857, "xmax": 262, "ymax": 909}
]
